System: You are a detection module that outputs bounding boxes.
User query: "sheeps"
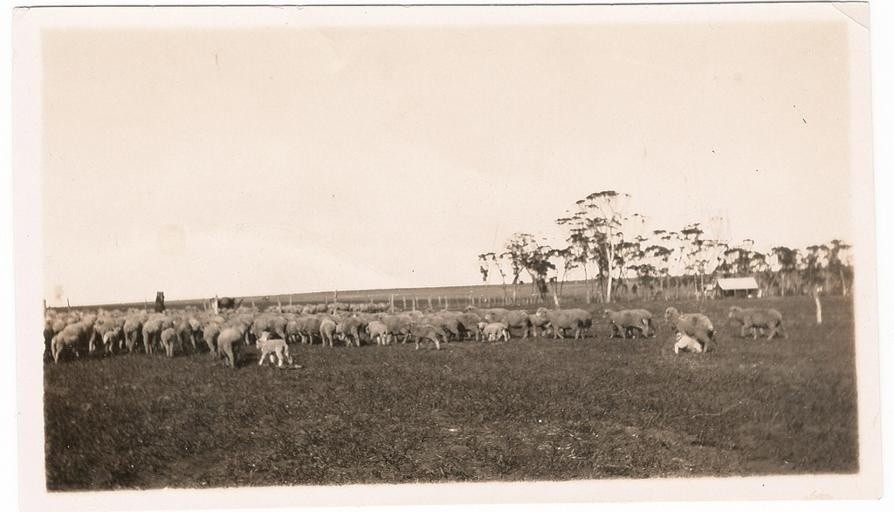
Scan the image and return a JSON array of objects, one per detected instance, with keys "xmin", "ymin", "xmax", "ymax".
[
  {"xmin": 44, "ymin": 301, "xmax": 593, "ymax": 368},
  {"xmin": 664, "ymin": 307, "xmax": 715, "ymax": 353},
  {"xmin": 602, "ymin": 308, "xmax": 656, "ymax": 340},
  {"xmin": 728, "ymin": 305, "xmax": 782, "ymax": 341}
]
